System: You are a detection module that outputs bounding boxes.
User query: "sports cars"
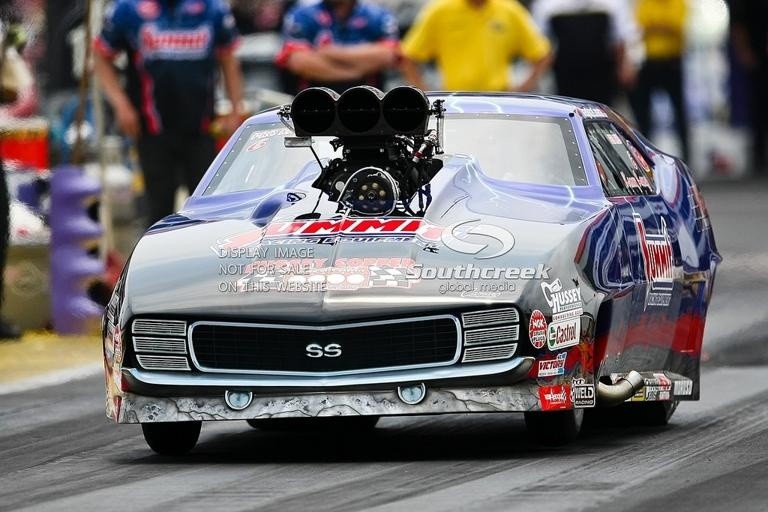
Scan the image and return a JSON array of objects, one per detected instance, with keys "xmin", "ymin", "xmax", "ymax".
[{"xmin": 99, "ymin": 83, "xmax": 722, "ymax": 447}]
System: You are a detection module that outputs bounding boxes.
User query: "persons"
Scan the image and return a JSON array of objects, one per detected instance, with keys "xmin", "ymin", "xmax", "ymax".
[
  {"xmin": 394, "ymin": 1, "xmax": 553, "ymax": 108},
  {"xmin": 273, "ymin": 0, "xmax": 401, "ymax": 94},
  {"xmin": 523, "ymin": 0, "xmax": 646, "ymax": 121},
  {"xmin": 88, "ymin": 0, "xmax": 245, "ymax": 223},
  {"xmin": 0, "ymin": 22, "xmax": 37, "ymax": 344},
  {"xmin": 617, "ymin": 0, "xmax": 701, "ymax": 168}
]
